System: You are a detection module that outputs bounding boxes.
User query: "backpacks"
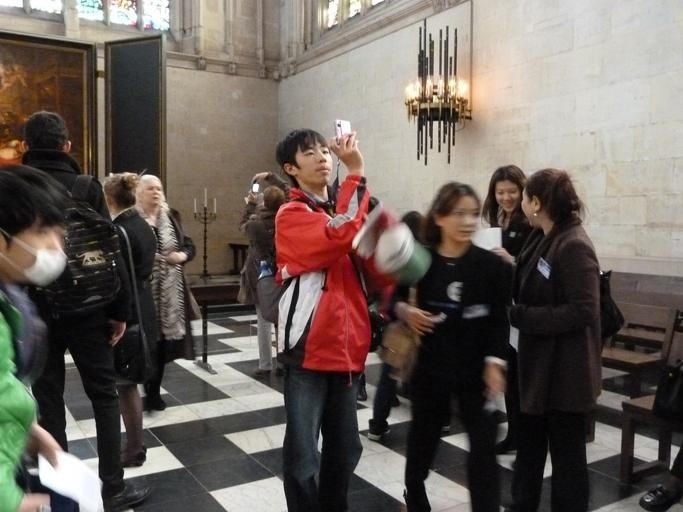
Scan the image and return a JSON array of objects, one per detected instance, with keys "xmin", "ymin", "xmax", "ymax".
[
  {"xmin": 256, "ymin": 252, "xmax": 291, "ymax": 322},
  {"xmin": 45, "ymin": 174, "xmax": 121, "ymax": 317}
]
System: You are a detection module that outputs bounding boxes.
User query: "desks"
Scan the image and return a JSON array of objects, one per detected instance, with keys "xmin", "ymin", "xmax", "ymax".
[{"xmin": 181, "ymin": 272, "xmax": 255, "ymax": 375}]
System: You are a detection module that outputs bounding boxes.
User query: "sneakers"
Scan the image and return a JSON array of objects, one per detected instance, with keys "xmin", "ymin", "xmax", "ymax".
[{"xmin": 367, "ymin": 420, "xmax": 391, "ymax": 440}]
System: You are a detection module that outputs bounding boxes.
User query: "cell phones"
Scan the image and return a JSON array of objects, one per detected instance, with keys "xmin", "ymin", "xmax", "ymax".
[
  {"xmin": 252, "ymin": 182, "xmax": 261, "ymax": 196},
  {"xmin": 335, "ymin": 117, "xmax": 353, "ymax": 145},
  {"xmin": 426, "ymin": 312, "xmax": 448, "ymax": 324}
]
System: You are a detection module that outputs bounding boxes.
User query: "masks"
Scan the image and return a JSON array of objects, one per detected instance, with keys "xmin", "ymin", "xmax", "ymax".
[{"xmin": 0, "ymin": 228, "xmax": 67, "ymax": 287}]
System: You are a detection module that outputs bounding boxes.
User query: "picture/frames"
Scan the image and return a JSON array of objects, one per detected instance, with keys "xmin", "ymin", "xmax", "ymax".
[{"xmin": 0, "ymin": 28, "xmax": 100, "ymax": 180}]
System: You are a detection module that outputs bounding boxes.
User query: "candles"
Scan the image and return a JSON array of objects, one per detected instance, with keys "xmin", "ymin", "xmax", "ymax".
[
  {"xmin": 213, "ymin": 198, "xmax": 218, "ymax": 213},
  {"xmin": 192, "ymin": 197, "xmax": 196, "ymax": 213},
  {"xmin": 203, "ymin": 188, "xmax": 208, "ymax": 208}
]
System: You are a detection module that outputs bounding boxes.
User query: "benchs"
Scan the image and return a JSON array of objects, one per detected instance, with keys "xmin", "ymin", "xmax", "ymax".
[{"xmin": 581, "ymin": 271, "xmax": 682, "ymax": 490}]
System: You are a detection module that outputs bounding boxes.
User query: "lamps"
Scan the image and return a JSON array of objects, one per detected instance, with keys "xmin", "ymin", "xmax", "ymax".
[{"xmin": 404, "ymin": 14, "xmax": 471, "ymax": 166}]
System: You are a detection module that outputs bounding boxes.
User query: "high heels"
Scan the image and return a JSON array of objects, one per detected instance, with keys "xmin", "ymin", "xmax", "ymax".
[
  {"xmin": 119, "ymin": 449, "xmax": 145, "ymax": 467},
  {"xmin": 146, "ymin": 393, "xmax": 166, "ymax": 413}
]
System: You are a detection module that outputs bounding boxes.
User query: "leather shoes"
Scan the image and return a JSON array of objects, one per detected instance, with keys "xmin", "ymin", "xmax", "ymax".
[
  {"xmin": 639, "ymin": 486, "xmax": 681, "ymax": 512},
  {"xmin": 105, "ymin": 484, "xmax": 152, "ymax": 511}
]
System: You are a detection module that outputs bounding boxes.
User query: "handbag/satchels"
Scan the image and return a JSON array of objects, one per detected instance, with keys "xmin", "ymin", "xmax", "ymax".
[
  {"xmin": 115, "ymin": 325, "xmax": 152, "ymax": 383},
  {"xmin": 599, "ymin": 269, "xmax": 624, "ymax": 336},
  {"xmin": 378, "ymin": 320, "xmax": 419, "ymax": 370}
]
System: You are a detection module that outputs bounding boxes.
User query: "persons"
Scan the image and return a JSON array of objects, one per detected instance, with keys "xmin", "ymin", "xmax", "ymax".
[
  {"xmin": 100, "ymin": 171, "xmax": 158, "ymax": 466},
  {"xmin": 19, "ymin": 112, "xmax": 152, "ymax": 510},
  {"xmin": 236, "ymin": 167, "xmax": 456, "ymax": 439},
  {"xmin": 637, "ymin": 360, "xmax": 681, "ymax": 510},
  {"xmin": 392, "ymin": 180, "xmax": 515, "ymax": 510},
  {"xmin": 512, "ymin": 169, "xmax": 605, "ymax": 510},
  {"xmin": 270, "ymin": 129, "xmax": 392, "ymax": 511},
  {"xmin": 137, "ymin": 175, "xmax": 202, "ymax": 411},
  {"xmin": 481, "ymin": 166, "xmax": 536, "ymax": 455},
  {"xmin": 1, "ymin": 164, "xmax": 75, "ymax": 511}
]
